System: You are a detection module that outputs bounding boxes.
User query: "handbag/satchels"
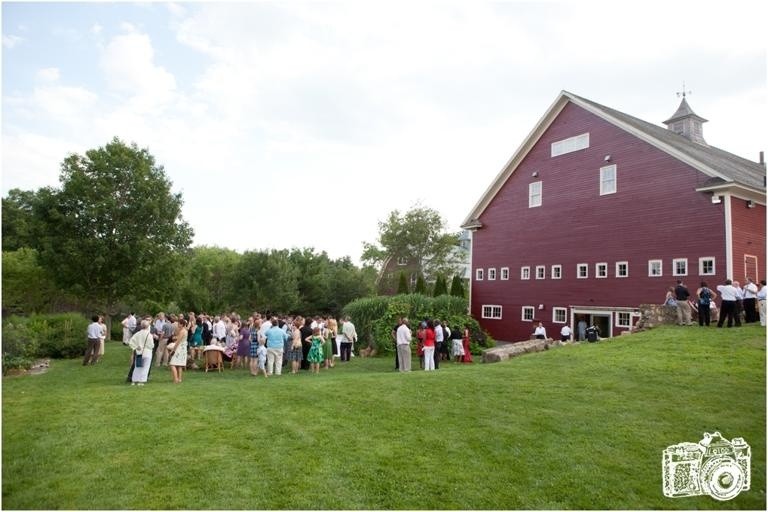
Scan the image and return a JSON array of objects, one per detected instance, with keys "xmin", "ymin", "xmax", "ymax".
[{"xmin": 136, "ymin": 354, "xmax": 142, "ymax": 367}]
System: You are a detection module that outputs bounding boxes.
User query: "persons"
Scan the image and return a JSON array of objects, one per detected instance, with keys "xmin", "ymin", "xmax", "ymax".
[
  {"xmin": 391, "ymin": 315, "xmax": 474, "ymax": 373},
  {"xmin": 80, "ymin": 308, "xmax": 359, "ymax": 387},
  {"xmin": 663, "ymin": 277, "xmax": 766, "ymax": 328},
  {"xmin": 530, "ymin": 317, "xmax": 599, "ymax": 342}
]
